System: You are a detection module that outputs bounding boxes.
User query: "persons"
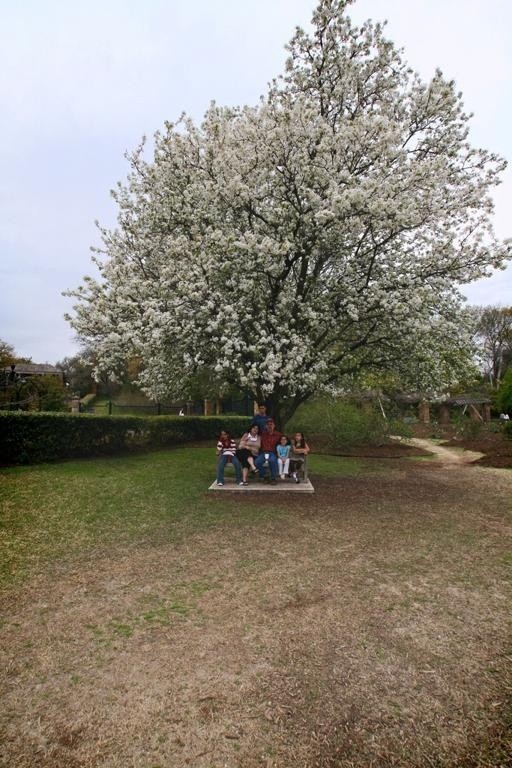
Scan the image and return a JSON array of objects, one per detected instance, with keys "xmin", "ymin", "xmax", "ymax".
[
  {"xmin": 256, "ymin": 420, "xmax": 286, "ymax": 485},
  {"xmin": 276, "ymin": 436, "xmax": 291, "ymax": 480},
  {"xmin": 289, "ymin": 432, "xmax": 310, "ymax": 483},
  {"xmin": 499, "ymin": 411, "xmax": 504, "ymax": 418},
  {"xmin": 250, "ymin": 403, "xmax": 272, "ymax": 433},
  {"xmin": 178, "ymin": 407, "xmax": 186, "ymax": 416},
  {"xmin": 217, "ymin": 429, "xmax": 243, "ymax": 486},
  {"xmin": 503, "ymin": 413, "xmax": 509, "ymax": 420},
  {"xmin": 237, "ymin": 424, "xmax": 262, "ymax": 484}
]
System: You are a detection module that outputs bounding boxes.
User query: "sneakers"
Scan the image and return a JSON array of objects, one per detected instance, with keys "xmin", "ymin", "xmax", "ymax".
[
  {"xmin": 237, "ymin": 481, "xmax": 249, "ymax": 486},
  {"xmin": 251, "ymin": 467, "xmax": 259, "ymax": 473},
  {"xmin": 218, "ymin": 482, "xmax": 223, "ymax": 486},
  {"xmin": 280, "ymin": 473, "xmax": 299, "ymax": 483},
  {"xmin": 264, "ymin": 476, "xmax": 277, "ymax": 485}
]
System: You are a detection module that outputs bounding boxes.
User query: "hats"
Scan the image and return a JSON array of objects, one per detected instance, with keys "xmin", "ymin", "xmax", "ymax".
[{"xmin": 265, "ymin": 418, "xmax": 273, "ymax": 423}]
